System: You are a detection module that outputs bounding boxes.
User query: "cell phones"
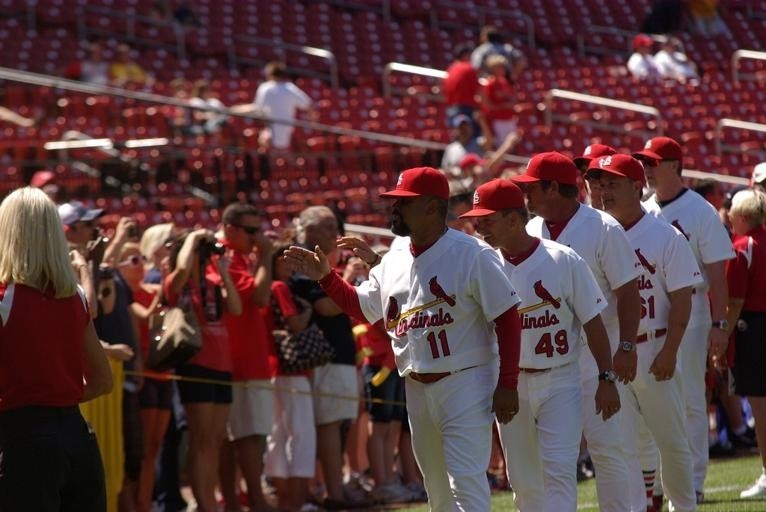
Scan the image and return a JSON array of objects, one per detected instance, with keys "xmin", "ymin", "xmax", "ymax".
[
  {"xmin": 89, "ymin": 236, "xmax": 105, "ymax": 264},
  {"xmin": 126, "ymin": 217, "xmax": 140, "ymax": 237}
]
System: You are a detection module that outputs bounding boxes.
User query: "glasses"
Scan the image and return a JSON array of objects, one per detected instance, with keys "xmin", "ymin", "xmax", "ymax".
[
  {"xmin": 116, "ymin": 254, "xmax": 149, "ymax": 269},
  {"xmin": 229, "ymin": 223, "xmax": 261, "ymax": 236}
]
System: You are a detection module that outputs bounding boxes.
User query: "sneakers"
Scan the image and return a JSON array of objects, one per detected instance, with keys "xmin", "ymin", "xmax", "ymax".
[{"xmin": 224, "ymin": 427, "xmax": 766, "ymax": 511}]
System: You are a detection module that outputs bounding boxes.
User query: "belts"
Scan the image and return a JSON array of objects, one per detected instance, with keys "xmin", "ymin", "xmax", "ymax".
[
  {"xmin": 407, "ymin": 372, "xmax": 449, "ymax": 384},
  {"xmin": 519, "ymin": 368, "xmax": 549, "ymax": 373},
  {"xmin": 636, "ymin": 328, "xmax": 667, "ymax": 344}
]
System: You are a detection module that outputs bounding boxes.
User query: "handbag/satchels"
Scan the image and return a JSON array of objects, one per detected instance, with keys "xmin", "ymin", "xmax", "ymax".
[
  {"xmin": 148, "ymin": 296, "xmax": 203, "ymax": 367},
  {"xmin": 271, "ymin": 324, "xmax": 337, "ymax": 375}
]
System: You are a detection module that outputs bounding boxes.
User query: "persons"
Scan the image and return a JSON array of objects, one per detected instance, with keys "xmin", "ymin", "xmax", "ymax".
[
  {"xmin": 107, "ymin": 45, "xmax": 155, "ymax": 98},
  {"xmin": 223, "ymin": 62, "xmax": 311, "ymax": 156},
  {"xmin": 165, "ymin": 81, "xmax": 232, "ymax": 140},
  {"xmin": 67, "ymin": 44, "xmax": 110, "ymax": 106},
  {"xmin": 443, "ymin": 27, "xmax": 530, "ymax": 155},
  {"xmin": 33, "ymin": 121, "xmax": 766, "ymax": 512},
  {"xmin": 0, "ymin": 186, "xmax": 114, "ymax": 512},
  {"xmin": 629, "ymin": 32, "xmax": 700, "ymax": 84}
]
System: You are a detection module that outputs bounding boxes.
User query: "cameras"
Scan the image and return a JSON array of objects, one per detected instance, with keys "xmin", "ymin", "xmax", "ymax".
[{"xmin": 199, "ymin": 233, "xmax": 226, "ymax": 262}]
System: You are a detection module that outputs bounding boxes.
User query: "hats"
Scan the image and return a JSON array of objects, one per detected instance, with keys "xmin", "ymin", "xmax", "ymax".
[
  {"xmin": 57, "ymin": 200, "xmax": 104, "ymax": 226},
  {"xmin": 752, "ymin": 162, "xmax": 766, "ymax": 188},
  {"xmin": 453, "ymin": 114, "xmax": 474, "ymax": 129},
  {"xmin": 378, "ymin": 137, "xmax": 683, "ymax": 220},
  {"xmin": 633, "ymin": 36, "xmax": 654, "ymax": 52},
  {"xmin": 29, "ymin": 168, "xmax": 60, "ymax": 190}
]
